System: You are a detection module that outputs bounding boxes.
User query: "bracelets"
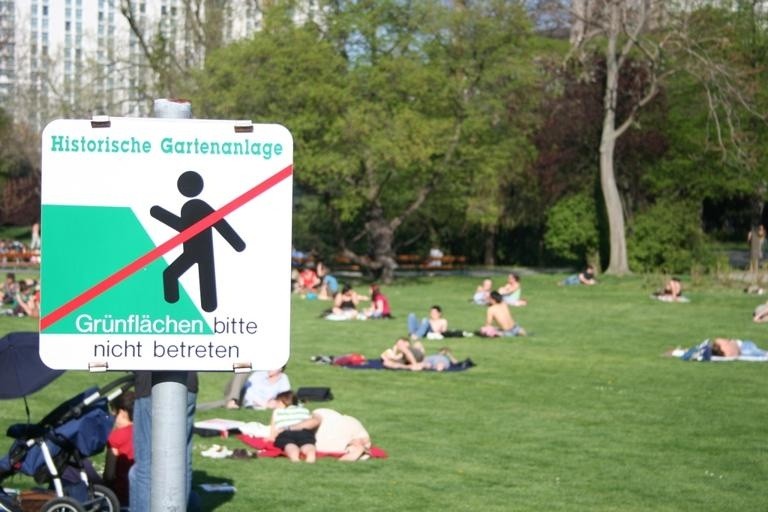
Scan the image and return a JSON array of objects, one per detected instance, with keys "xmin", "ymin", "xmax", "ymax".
[{"xmin": 287, "ymin": 426, "xmax": 290, "ymax": 431}]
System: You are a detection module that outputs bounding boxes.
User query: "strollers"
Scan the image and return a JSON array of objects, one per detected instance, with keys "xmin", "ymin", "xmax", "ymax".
[{"xmin": 0, "ymin": 374, "xmax": 135, "ymax": 511}]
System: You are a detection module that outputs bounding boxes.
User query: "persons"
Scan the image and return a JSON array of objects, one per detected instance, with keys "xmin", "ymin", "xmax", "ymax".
[
  {"xmin": 753, "ymin": 301, "xmax": 768, "ymax": 322},
  {"xmin": 744, "ymin": 223, "xmax": 765, "ymax": 271},
  {"xmin": 368, "ymin": 265, "xmax": 385, "ymax": 285},
  {"xmin": 557, "ymin": 266, "xmax": 595, "ymax": 287},
  {"xmin": 475, "ymin": 279, "xmax": 493, "ymax": 305},
  {"xmin": 498, "ymin": 272, "xmax": 526, "ymax": 306},
  {"xmin": 744, "ymin": 286, "xmax": 764, "ymax": 294},
  {"xmin": 331, "ymin": 284, "xmax": 368, "ymax": 315},
  {"xmin": 196, "ymin": 365, "xmax": 290, "ymax": 411},
  {"xmin": 660, "ymin": 277, "xmax": 686, "ymax": 300},
  {"xmin": 291, "ymin": 262, "xmax": 340, "ymax": 299},
  {"xmin": 408, "ymin": 305, "xmax": 448, "ymax": 340},
  {"xmin": 709, "ymin": 338, "xmax": 768, "ymax": 357},
  {"xmin": 270, "ymin": 391, "xmax": 317, "ymax": 464},
  {"xmin": 487, "ymin": 291, "xmax": 526, "ymax": 337},
  {"xmin": 381, "ymin": 337, "xmax": 458, "ymax": 371},
  {"xmin": 129, "ymin": 371, "xmax": 199, "ymax": 512},
  {"xmin": 364, "ymin": 283, "xmax": 391, "ymax": 318},
  {"xmin": 107, "ymin": 390, "xmax": 134, "ymax": 459},
  {"xmin": 274, "ymin": 408, "xmax": 371, "ymax": 461},
  {"xmin": 0, "ymin": 225, "xmax": 40, "ymax": 319}
]
